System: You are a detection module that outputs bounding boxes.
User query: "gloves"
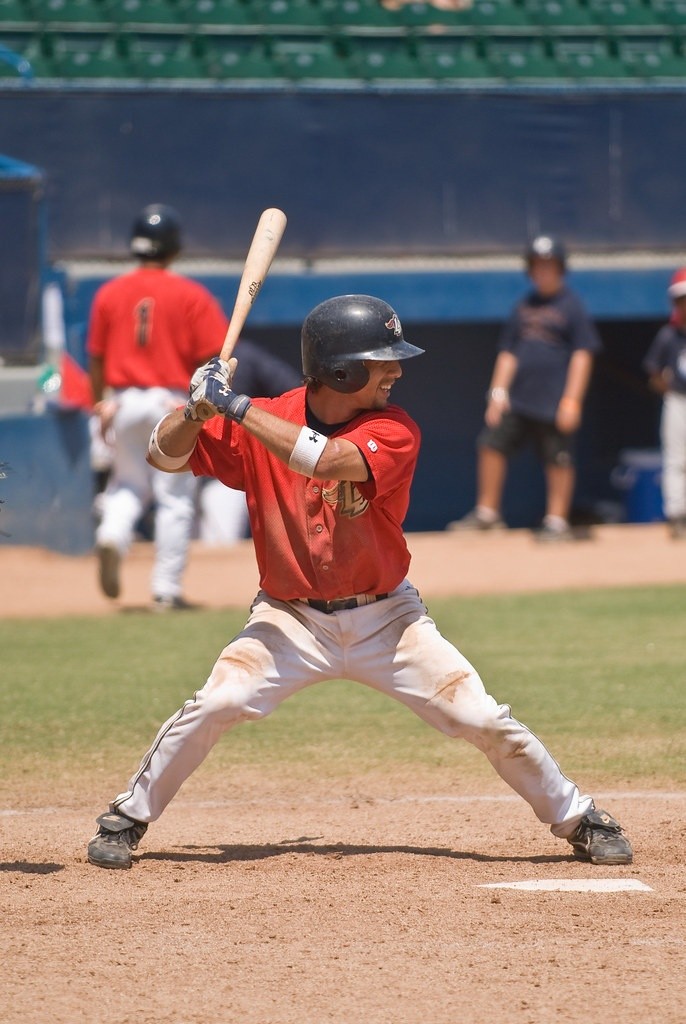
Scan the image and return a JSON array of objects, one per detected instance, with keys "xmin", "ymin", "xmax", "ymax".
[
  {"xmin": 189, "ymin": 358, "xmax": 230, "ymax": 395},
  {"xmin": 185, "ymin": 377, "xmax": 252, "ymax": 425}
]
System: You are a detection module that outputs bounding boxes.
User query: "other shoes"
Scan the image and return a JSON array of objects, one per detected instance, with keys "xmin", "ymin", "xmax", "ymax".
[
  {"xmin": 99, "ymin": 545, "xmax": 119, "ymax": 598},
  {"xmin": 537, "ymin": 523, "xmax": 571, "ymax": 542},
  {"xmin": 447, "ymin": 511, "xmax": 499, "ymax": 529},
  {"xmin": 154, "ymin": 598, "xmax": 196, "ymax": 609}
]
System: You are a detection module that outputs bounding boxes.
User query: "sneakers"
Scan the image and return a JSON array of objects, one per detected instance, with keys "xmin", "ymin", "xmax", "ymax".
[
  {"xmin": 88, "ymin": 811, "xmax": 149, "ymax": 869},
  {"xmin": 568, "ymin": 811, "xmax": 633, "ymax": 864}
]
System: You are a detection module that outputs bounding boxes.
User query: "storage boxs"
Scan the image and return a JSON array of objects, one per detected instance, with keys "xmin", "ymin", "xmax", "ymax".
[{"xmin": 618, "ymin": 449, "xmax": 668, "ymax": 522}]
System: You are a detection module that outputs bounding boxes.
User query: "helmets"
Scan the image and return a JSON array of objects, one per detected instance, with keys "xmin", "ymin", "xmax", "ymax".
[
  {"xmin": 127, "ymin": 206, "xmax": 181, "ymax": 260},
  {"xmin": 301, "ymin": 295, "xmax": 426, "ymax": 394},
  {"xmin": 524, "ymin": 235, "xmax": 569, "ymax": 275}
]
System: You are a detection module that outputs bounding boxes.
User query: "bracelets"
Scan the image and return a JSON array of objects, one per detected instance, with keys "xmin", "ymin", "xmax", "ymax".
[{"xmin": 488, "ymin": 389, "xmax": 506, "ymax": 398}]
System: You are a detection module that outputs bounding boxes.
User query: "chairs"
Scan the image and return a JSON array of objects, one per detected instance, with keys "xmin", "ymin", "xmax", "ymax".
[{"xmin": 0, "ymin": 0, "xmax": 686, "ymax": 79}]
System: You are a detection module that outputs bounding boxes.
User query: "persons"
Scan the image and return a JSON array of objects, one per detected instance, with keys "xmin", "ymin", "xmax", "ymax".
[
  {"xmin": 86, "ymin": 202, "xmax": 305, "ymax": 616},
  {"xmin": 644, "ymin": 267, "xmax": 686, "ymax": 540},
  {"xmin": 88, "ymin": 296, "xmax": 635, "ymax": 870},
  {"xmin": 445, "ymin": 232, "xmax": 604, "ymax": 545}
]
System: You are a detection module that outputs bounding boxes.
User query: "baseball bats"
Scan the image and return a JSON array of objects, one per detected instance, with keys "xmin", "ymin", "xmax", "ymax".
[{"xmin": 194, "ymin": 208, "xmax": 289, "ymax": 420}]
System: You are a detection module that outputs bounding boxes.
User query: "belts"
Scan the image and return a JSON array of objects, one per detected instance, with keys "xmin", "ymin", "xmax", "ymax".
[{"xmin": 292, "ymin": 593, "xmax": 388, "ymax": 614}]
